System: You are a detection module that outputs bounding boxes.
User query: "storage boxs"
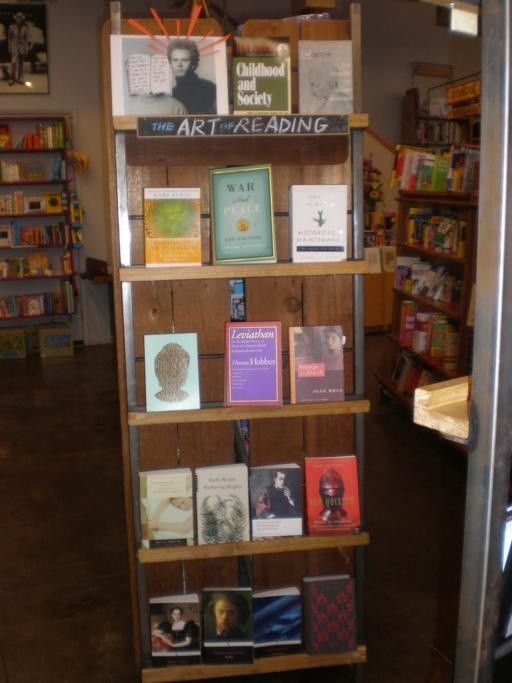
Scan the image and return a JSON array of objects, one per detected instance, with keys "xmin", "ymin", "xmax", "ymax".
[
  {"xmin": 1, "ymin": 325, "xmax": 29, "ymax": 362},
  {"xmin": 34, "ymin": 321, "xmax": 75, "ymax": 360}
]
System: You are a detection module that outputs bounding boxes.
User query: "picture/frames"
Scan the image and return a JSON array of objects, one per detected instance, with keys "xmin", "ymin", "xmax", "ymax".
[{"xmin": 1, "ymin": 1, "xmax": 52, "ymax": 97}]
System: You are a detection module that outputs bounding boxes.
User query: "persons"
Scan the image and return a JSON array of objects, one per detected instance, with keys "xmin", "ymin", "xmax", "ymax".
[{"xmin": 0, "ymin": 12, "xmax": 45, "ymax": 86}]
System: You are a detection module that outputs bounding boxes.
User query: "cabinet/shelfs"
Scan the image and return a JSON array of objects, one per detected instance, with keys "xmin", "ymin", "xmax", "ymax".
[
  {"xmin": 1, "ymin": 109, "xmax": 85, "ymax": 326},
  {"xmin": 102, "ymin": 1, "xmax": 377, "ymax": 682},
  {"xmin": 365, "ymin": 67, "xmax": 484, "ymax": 438}
]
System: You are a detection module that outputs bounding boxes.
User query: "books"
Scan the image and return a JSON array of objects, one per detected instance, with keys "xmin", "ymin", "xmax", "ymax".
[
  {"xmin": 224, "ymin": 321, "xmax": 282, "ymax": 407},
  {"xmin": 144, "ymin": 333, "xmax": 200, "ymax": 411},
  {"xmin": 139, "ymin": 455, "xmax": 362, "ymax": 550},
  {"xmin": 390, "ymin": 119, "xmax": 479, "ymax": 402},
  {"xmin": 143, "ymin": 187, "xmax": 202, "ymax": 267},
  {"xmin": 210, "ymin": 163, "xmax": 277, "ymax": 266},
  {"xmin": 289, "ymin": 326, "xmax": 345, "ymax": 404},
  {"xmin": 148, "ymin": 574, "xmax": 358, "ymax": 666},
  {"xmin": 289, "ymin": 185, "xmax": 347, "ymax": 262},
  {"xmin": 0, "ymin": 121, "xmax": 77, "ymax": 359},
  {"xmin": 110, "ymin": 31, "xmax": 363, "ymax": 115}
]
